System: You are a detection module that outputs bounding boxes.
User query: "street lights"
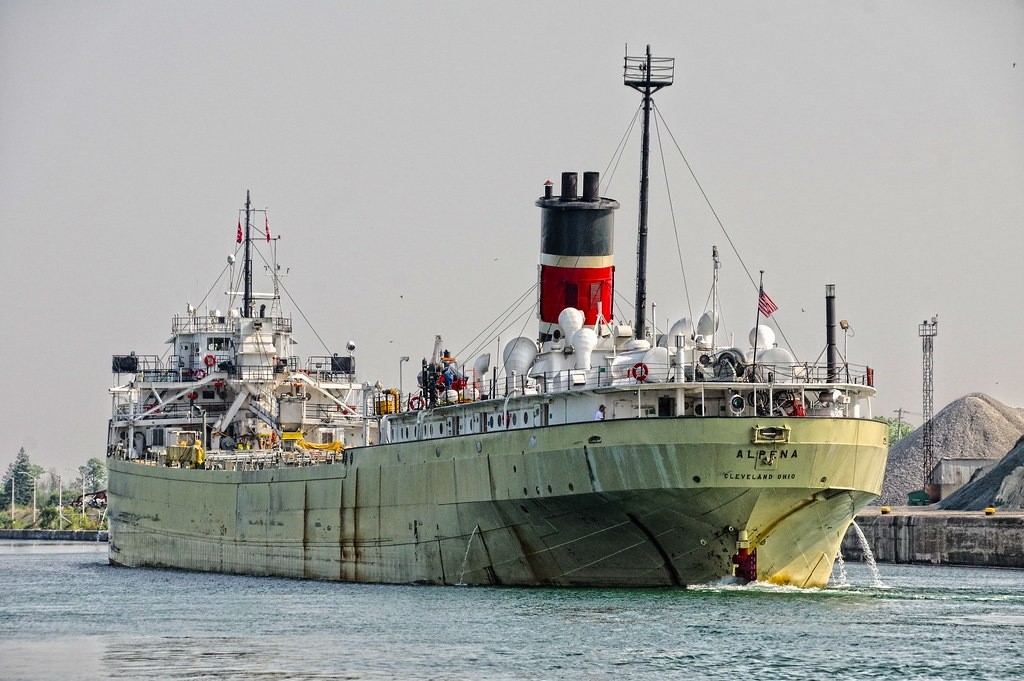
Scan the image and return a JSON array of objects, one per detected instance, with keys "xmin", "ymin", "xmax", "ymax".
[
  {"xmin": 45, "ymin": 470, "xmax": 63, "ymax": 530},
  {"xmin": 67, "ymin": 468, "xmax": 88, "ymax": 528},
  {"xmin": 16, "ymin": 472, "xmax": 39, "ymax": 528},
  {"xmin": 6, "ymin": 475, "xmax": 18, "ymax": 528}
]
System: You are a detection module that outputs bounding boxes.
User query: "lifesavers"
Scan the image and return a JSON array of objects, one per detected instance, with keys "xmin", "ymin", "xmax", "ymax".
[
  {"xmin": 205, "ymin": 354, "xmax": 216, "ymax": 366},
  {"xmin": 195, "ymin": 369, "xmax": 206, "ymax": 380},
  {"xmin": 147, "ymin": 405, "xmax": 159, "ymax": 414},
  {"xmin": 411, "ymin": 397, "xmax": 425, "ymax": 410},
  {"xmin": 633, "ymin": 363, "xmax": 648, "ymax": 380}
]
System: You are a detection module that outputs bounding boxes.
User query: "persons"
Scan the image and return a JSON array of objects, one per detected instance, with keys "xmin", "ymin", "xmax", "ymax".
[
  {"xmin": 117, "ymin": 440, "xmax": 124, "ymax": 458},
  {"xmin": 788, "ymin": 399, "xmax": 807, "ymax": 417},
  {"xmin": 593, "ymin": 404, "xmax": 607, "ymax": 421}
]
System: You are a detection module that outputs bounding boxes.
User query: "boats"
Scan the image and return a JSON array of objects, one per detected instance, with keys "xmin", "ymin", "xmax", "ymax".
[{"xmin": 103, "ymin": 42, "xmax": 894, "ymax": 597}]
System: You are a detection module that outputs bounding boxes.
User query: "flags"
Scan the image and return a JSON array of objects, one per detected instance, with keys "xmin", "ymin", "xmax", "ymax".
[{"xmin": 758, "ymin": 281, "xmax": 779, "ymax": 317}]
